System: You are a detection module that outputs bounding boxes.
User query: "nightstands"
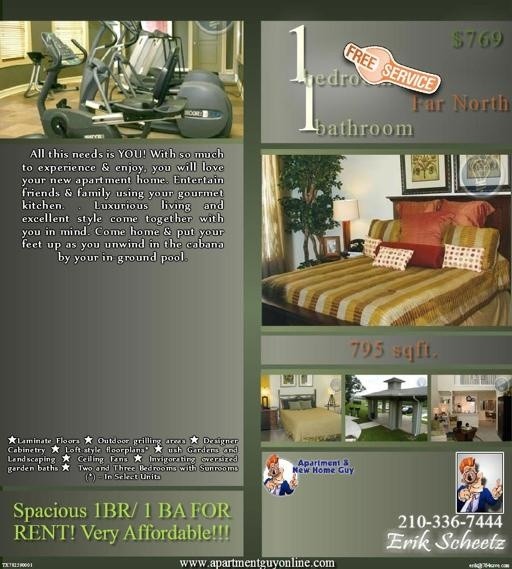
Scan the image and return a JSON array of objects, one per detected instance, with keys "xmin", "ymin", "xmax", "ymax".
[{"xmin": 261, "ymin": 407, "xmax": 279, "ymax": 431}]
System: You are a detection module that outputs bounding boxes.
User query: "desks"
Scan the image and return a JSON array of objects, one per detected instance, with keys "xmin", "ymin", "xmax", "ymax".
[
  {"xmin": 324, "ymin": 403, "xmax": 340, "ymax": 412},
  {"xmin": 453, "ymin": 426, "xmax": 477, "ymax": 441}
]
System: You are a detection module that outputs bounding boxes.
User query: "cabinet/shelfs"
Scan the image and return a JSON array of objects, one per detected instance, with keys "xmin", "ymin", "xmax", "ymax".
[{"xmin": 497, "ymin": 395, "xmax": 512, "ymax": 441}]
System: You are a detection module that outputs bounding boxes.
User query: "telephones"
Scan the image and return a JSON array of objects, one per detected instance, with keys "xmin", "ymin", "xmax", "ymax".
[{"xmin": 349, "ymin": 239, "xmax": 365, "ymax": 252}]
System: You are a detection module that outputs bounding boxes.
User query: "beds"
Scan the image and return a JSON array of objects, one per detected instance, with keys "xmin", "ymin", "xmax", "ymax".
[
  {"xmin": 277, "ymin": 388, "xmax": 341, "ymax": 443},
  {"xmin": 262, "ymin": 194, "xmax": 510, "ymax": 327}
]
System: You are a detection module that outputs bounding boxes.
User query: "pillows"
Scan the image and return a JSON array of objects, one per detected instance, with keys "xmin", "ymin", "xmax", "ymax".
[
  {"xmin": 288, "ymin": 399, "xmax": 312, "ymax": 411},
  {"xmin": 362, "ymin": 199, "xmax": 502, "ymax": 274}
]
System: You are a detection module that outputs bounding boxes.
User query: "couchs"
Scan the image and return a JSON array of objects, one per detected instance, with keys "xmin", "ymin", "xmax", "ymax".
[{"xmin": 430, "ymin": 419, "xmax": 448, "ymax": 441}]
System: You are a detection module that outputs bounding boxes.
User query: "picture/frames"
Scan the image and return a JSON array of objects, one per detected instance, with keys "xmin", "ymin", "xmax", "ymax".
[
  {"xmin": 399, "ymin": 151, "xmax": 511, "ymax": 195},
  {"xmin": 322, "ymin": 236, "xmax": 340, "ymax": 258},
  {"xmin": 280, "ymin": 374, "xmax": 313, "ymax": 387}
]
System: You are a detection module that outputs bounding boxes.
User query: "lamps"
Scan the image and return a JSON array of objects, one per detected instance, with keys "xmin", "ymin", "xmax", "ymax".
[{"xmin": 333, "ymin": 198, "xmax": 358, "ymax": 256}]
